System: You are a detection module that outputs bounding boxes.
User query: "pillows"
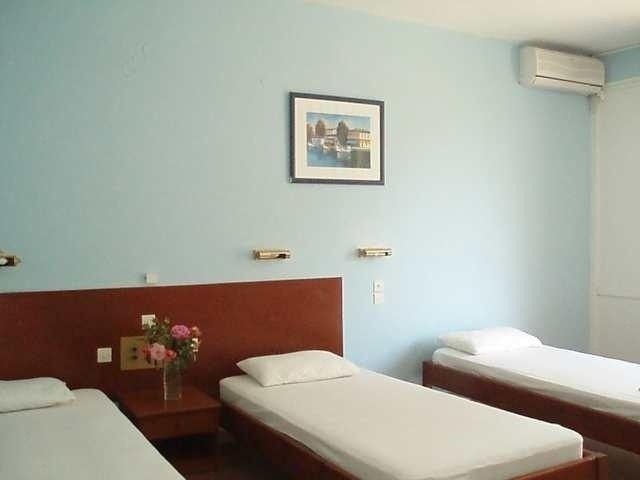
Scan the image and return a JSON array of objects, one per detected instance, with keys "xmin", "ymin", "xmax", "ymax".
[
  {"xmin": 236, "ymin": 350, "xmax": 353, "ymax": 387},
  {"xmin": 0, "ymin": 377, "xmax": 76, "ymax": 414},
  {"xmin": 437, "ymin": 326, "xmax": 543, "ymax": 355}
]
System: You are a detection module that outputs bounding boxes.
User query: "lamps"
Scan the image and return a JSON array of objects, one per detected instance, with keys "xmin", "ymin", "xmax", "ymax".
[
  {"xmin": 0, "ymin": 252, "xmax": 21, "ymax": 268},
  {"xmin": 252, "ymin": 248, "xmax": 291, "ymax": 261},
  {"xmin": 358, "ymin": 249, "xmax": 392, "ymax": 257}
]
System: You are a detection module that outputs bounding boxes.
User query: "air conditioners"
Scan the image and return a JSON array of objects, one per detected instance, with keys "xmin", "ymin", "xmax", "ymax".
[{"xmin": 519, "ymin": 46, "xmax": 605, "ymax": 97}]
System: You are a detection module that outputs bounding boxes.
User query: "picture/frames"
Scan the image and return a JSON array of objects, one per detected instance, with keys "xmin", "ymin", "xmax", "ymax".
[{"xmin": 289, "ymin": 92, "xmax": 385, "ymax": 186}]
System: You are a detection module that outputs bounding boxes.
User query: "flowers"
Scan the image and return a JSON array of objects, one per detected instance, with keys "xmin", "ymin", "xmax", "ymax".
[{"xmin": 142, "ymin": 314, "xmax": 203, "ymax": 371}]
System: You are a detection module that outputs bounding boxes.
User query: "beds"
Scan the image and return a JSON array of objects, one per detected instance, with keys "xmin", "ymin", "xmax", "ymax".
[
  {"xmin": 422, "ymin": 341, "xmax": 639, "ymax": 479},
  {"xmin": 218, "ymin": 359, "xmax": 608, "ymax": 480},
  {"xmin": 0, "ymin": 388, "xmax": 185, "ymax": 480}
]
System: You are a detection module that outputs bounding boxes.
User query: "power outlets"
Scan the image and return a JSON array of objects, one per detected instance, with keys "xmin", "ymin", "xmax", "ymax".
[{"xmin": 121, "ymin": 336, "xmax": 163, "ymax": 371}]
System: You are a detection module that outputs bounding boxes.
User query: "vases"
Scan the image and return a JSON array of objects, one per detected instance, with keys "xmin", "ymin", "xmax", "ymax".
[{"xmin": 162, "ymin": 357, "xmax": 182, "ymax": 400}]
